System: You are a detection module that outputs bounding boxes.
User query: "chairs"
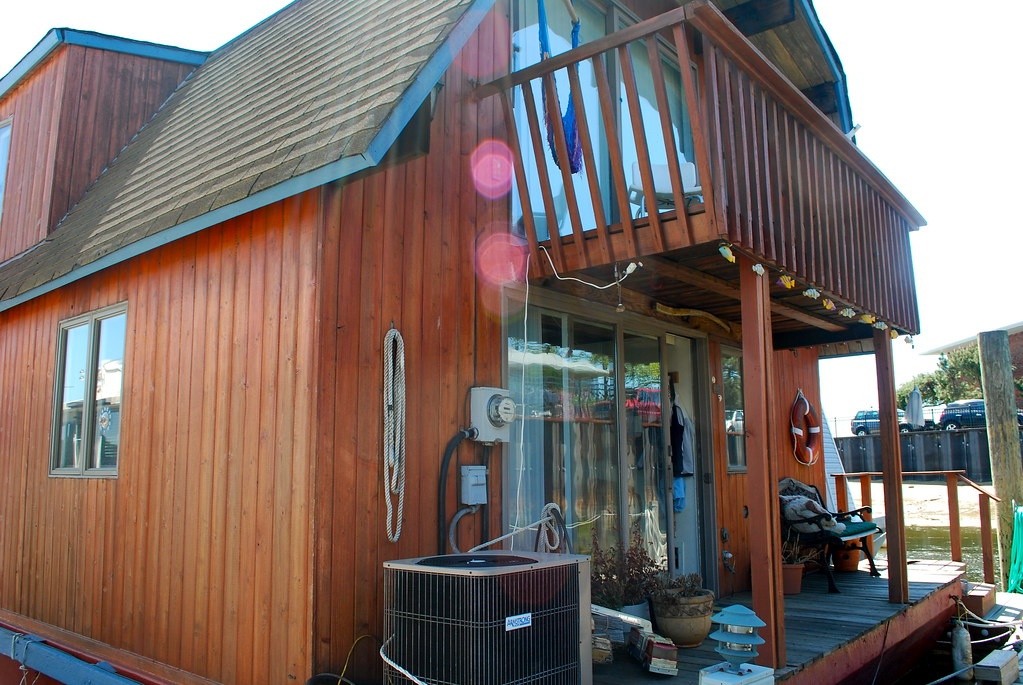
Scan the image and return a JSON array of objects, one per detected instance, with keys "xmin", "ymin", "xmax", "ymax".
[{"xmin": 776, "ymin": 478, "xmax": 884, "ymax": 593}]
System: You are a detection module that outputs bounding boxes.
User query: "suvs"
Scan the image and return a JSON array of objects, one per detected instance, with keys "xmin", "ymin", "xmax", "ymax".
[
  {"xmin": 592, "ymin": 386, "xmax": 661, "ymax": 425},
  {"xmin": 938, "ymin": 397, "xmax": 1023, "ymax": 432},
  {"xmin": 850, "ymin": 406, "xmax": 914, "ymax": 437}
]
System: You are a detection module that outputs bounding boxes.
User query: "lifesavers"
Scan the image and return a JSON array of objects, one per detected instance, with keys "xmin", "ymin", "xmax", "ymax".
[{"xmin": 791, "ymin": 398, "xmax": 820, "ymax": 464}]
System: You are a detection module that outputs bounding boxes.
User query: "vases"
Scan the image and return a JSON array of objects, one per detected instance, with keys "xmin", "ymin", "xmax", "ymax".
[{"xmin": 831, "ymin": 535, "xmax": 861, "ymax": 572}]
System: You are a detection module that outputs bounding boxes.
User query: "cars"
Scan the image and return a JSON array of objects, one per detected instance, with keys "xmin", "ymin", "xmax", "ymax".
[
  {"xmin": 921, "ymin": 403, "xmax": 945, "ymax": 431},
  {"xmin": 725, "ymin": 407, "xmax": 744, "ymax": 436}
]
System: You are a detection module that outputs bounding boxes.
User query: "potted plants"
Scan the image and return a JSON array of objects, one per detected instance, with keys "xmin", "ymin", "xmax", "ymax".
[
  {"xmin": 781, "ymin": 527, "xmax": 824, "ymax": 595},
  {"xmin": 588, "ymin": 518, "xmax": 715, "ymax": 649}
]
